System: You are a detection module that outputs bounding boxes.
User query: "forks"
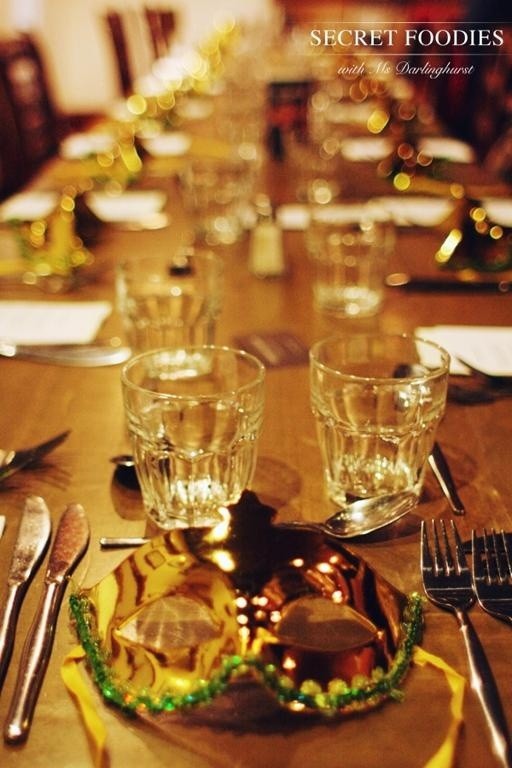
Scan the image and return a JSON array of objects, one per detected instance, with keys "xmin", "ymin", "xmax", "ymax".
[
  {"xmin": 0, "ymin": 429, "xmax": 73, "ymax": 488},
  {"xmin": 414, "ymin": 510, "xmax": 511, "ymax": 768}
]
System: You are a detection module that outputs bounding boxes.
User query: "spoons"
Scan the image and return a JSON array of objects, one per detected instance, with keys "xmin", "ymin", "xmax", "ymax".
[
  {"xmin": 94, "ymin": 488, "xmax": 423, "ymax": 553},
  {"xmin": 386, "ymin": 361, "xmax": 472, "ymax": 520}
]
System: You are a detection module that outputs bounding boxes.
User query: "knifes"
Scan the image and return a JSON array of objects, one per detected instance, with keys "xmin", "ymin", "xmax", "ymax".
[
  {"xmin": 8, "ymin": 494, "xmax": 93, "ymax": 751},
  {"xmin": 1, "ymin": 491, "xmax": 56, "ymax": 695},
  {"xmin": 0, "ymin": 344, "xmax": 131, "ymax": 369}
]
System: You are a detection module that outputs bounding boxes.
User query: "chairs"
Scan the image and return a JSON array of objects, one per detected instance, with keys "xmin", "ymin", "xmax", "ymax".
[
  {"xmin": 0, "ymin": 32, "xmax": 108, "ymax": 207},
  {"xmin": 102, "ymin": 4, "xmax": 179, "ymax": 103}
]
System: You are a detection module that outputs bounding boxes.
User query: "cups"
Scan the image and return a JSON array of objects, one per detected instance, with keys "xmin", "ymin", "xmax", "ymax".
[
  {"xmin": 119, "ymin": 342, "xmax": 270, "ymax": 530},
  {"xmin": 116, "ymin": 92, "xmax": 401, "ymax": 366},
  {"xmin": 307, "ymin": 327, "xmax": 450, "ymax": 504}
]
